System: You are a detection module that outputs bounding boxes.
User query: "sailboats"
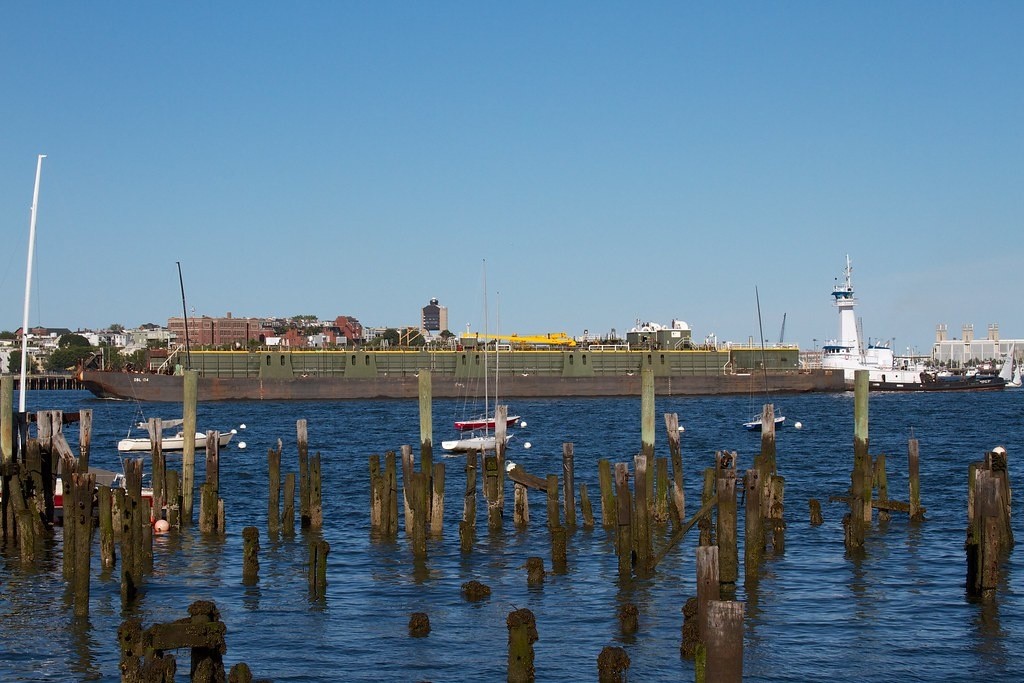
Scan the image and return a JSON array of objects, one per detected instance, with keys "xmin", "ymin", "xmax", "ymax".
[
  {"xmin": 742, "ymin": 286, "xmax": 786, "ymax": 431},
  {"xmin": 997, "ymin": 342, "xmax": 1022, "ymax": 388},
  {"xmin": 441, "ymin": 291, "xmax": 514, "ymax": 452},
  {"xmin": 0, "ymin": 156, "xmax": 175, "ymax": 521},
  {"xmin": 453, "ymin": 260, "xmax": 522, "ymax": 429}
]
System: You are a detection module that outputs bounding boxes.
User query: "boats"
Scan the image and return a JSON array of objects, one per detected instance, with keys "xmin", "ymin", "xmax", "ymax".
[
  {"xmin": 118, "ymin": 430, "xmax": 238, "ymax": 452},
  {"xmin": 846, "ymin": 370, "xmax": 1008, "ymax": 392},
  {"xmin": 78, "ymin": 262, "xmax": 845, "ymax": 402}
]
